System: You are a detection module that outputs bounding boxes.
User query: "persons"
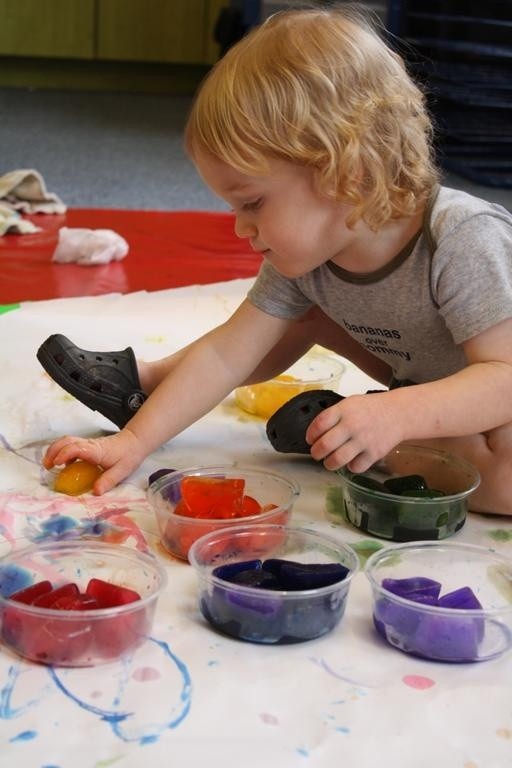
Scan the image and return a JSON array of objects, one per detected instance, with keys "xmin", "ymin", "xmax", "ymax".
[{"xmin": 37, "ymin": 6, "xmax": 512, "ymax": 518}]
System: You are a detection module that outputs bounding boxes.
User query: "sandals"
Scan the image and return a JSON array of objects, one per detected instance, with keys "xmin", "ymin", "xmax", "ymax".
[
  {"xmin": 37, "ymin": 335, "xmax": 149, "ymax": 430},
  {"xmin": 266, "ymin": 390, "xmax": 388, "ymax": 453}
]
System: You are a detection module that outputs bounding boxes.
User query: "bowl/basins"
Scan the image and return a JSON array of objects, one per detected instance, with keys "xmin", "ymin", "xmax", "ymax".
[
  {"xmin": 364, "ymin": 540, "xmax": 511, "ymax": 664},
  {"xmin": 147, "ymin": 463, "xmax": 302, "ymax": 564},
  {"xmin": 234, "ymin": 355, "xmax": 346, "ymax": 420},
  {"xmin": 1, "ymin": 541, "xmax": 168, "ymax": 669},
  {"xmin": 186, "ymin": 521, "xmax": 361, "ymax": 646},
  {"xmin": 336, "ymin": 448, "xmax": 482, "ymax": 542}
]
